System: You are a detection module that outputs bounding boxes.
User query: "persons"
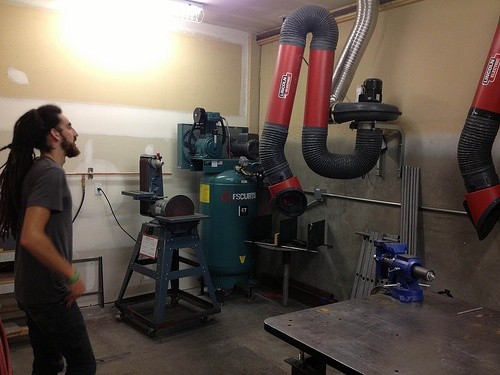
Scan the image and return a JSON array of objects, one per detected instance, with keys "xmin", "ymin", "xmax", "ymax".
[{"xmin": 0, "ymin": 102, "xmax": 97, "ymax": 375}]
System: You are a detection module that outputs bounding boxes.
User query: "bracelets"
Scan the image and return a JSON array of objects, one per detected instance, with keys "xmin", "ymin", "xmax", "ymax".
[{"xmin": 69, "ymin": 271, "xmax": 80, "ymax": 283}]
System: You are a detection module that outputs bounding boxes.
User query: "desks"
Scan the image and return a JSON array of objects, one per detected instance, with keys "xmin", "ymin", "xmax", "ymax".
[{"xmin": 263, "ymin": 291, "xmax": 500, "ymax": 375}]
